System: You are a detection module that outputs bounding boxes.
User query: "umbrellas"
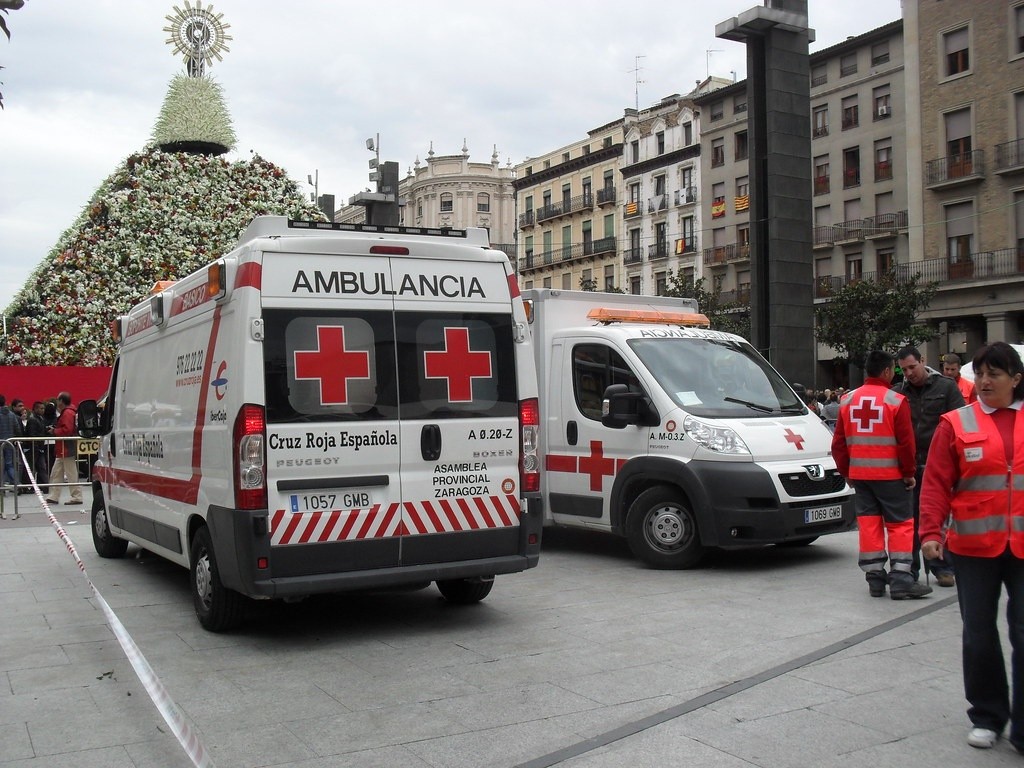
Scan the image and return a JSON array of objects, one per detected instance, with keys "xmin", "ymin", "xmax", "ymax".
[{"xmin": 958, "ymin": 344, "xmax": 1024, "ymax": 386}]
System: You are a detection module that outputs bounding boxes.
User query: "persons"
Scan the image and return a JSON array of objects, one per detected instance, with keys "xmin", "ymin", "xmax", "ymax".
[
  {"xmin": 830, "ymin": 349, "xmax": 933, "ymax": 601},
  {"xmin": 807, "ymin": 387, "xmax": 852, "ymax": 431},
  {"xmin": 45, "ymin": 390, "xmax": 84, "ymax": 505},
  {"xmin": 0, "ymin": 395, "xmax": 62, "ymax": 496},
  {"xmin": 893, "ymin": 345, "xmax": 966, "ymax": 589},
  {"xmin": 917, "ymin": 340, "xmax": 1024, "ymax": 757},
  {"xmin": 940, "ymin": 349, "xmax": 978, "ymax": 407}
]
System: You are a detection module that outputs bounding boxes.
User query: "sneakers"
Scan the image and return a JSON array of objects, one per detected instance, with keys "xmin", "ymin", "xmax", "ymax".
[
  {"xmin": 936, "ymin": 573, "xmax": 954, "ymax": 586},
  {"xmin": 967, "ymin": 727, "xmax": 998, "ymax": 747},
  {"xmin": 890, "ymin": 582, "xmax": 933, "ymax": 599},
  {"xmin": 869, "ymin": 584, "xmax": 886, "ymax": 597}
]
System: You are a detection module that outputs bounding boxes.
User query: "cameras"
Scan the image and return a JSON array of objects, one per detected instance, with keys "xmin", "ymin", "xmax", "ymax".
[{"xmin": 46, "ymin": 425, "xmax": 56, "ymax": 432}]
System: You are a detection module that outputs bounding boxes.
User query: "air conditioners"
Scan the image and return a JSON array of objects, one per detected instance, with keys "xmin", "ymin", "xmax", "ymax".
[{"xmin": 879, "ymin": 106, "xmax": 891, "ymax": 116}]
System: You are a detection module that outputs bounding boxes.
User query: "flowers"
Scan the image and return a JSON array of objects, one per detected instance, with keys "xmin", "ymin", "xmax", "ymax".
[{"xmin": 0, "ymin": 141, "xmax": 332, "ymax": 368}]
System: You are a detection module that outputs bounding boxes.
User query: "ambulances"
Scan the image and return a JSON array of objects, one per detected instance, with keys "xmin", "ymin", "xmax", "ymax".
[
  {"xmin": 72, "ymin": 213, "xmax": 549, "ymax": 638},
  {"xmin": 520, "ymin": 287, "xmax": 857, "ymax": 571}
]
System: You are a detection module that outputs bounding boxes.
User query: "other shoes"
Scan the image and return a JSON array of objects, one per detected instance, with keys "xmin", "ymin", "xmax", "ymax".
[
  {"xmin": 46, "ymin": 499, "xmax": 58, "ymax": 504},
  {"xmin": 18, "ymin": 487, "xmax": 22, "ymax": 496},
  {"xmin": 65, "ymin": 501, "xmax": 82, "ymax": 505}
]
